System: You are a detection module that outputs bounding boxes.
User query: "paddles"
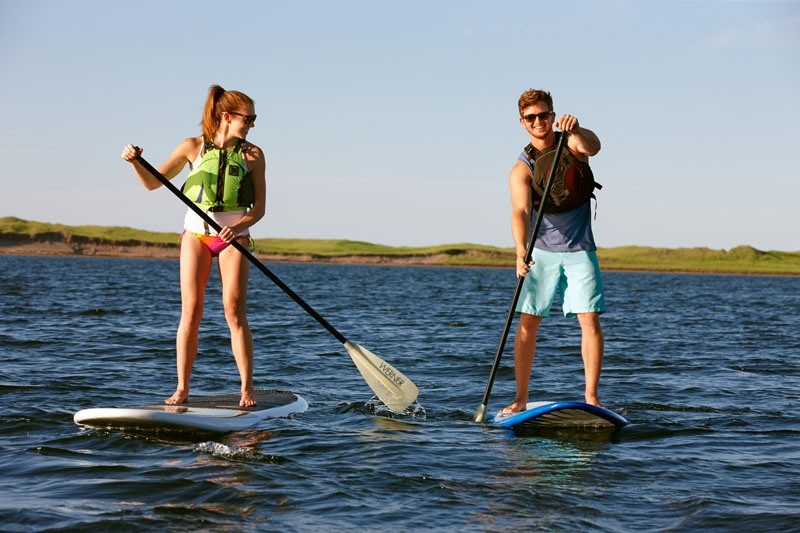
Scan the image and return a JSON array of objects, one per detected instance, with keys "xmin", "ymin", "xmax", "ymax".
[
  {"xmin": 473, "ymin": 125, "xmax": 570, "ymax": 424},
  {"xmin": 126, "ymin": 146, "xmax": 419, "ymax": 414}
]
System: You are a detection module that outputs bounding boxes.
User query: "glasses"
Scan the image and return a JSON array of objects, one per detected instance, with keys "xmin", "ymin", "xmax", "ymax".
[
  {"xmin": 523, "ymin": 110, "xmax": 553, "ymax": 123},
  {"xmin": 220, "ymin": 111, "xmax": 257, "ymax": 124}
]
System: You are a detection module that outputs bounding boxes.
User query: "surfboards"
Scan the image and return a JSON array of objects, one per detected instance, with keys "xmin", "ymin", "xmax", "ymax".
[
  {"xmin": 493, "ymin": 399, "xmax": 629, "ymax": 428},
  {"xmin": 73, "ymin": 389, "xmax": 309, "ymax": 431}
]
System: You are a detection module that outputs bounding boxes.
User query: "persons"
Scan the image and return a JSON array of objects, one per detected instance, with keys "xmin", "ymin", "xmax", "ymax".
[
  {"xmin": 498, "ymin": 89, "xmax": 608, "ymax": 415},
  {"xmin": 122, "ymin": 85, "xmax": 266, "ymax": 407}
]
